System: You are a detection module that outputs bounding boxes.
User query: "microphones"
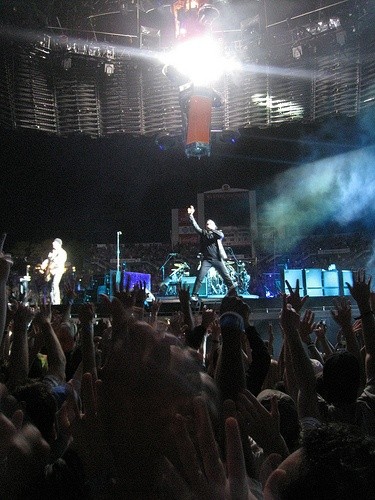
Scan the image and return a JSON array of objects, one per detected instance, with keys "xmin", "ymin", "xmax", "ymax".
[{"xmin": 118, "ymin": 231, "xmax": 123, "ymax": 235}]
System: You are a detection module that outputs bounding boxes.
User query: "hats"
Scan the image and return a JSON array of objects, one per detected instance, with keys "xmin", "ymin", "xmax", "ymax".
[
  {"xmin": 257, "ymin": 389, "xmax": 297, "ymax": 425},
  {"xmin": 55, "ymin": 238, "xmax": 62, "ymax": 245}
]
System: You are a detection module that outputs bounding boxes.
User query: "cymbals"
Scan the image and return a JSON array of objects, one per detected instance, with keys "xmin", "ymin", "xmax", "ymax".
[
  {"xmin": 174, "ymin": 262, "xmax": 189, "ymax": 268},
  {"xmin": 224, "ymin": 260, "xmax": 235, "ymax": 265}
]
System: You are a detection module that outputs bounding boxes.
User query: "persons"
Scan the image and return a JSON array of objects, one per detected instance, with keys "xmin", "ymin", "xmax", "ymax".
[
  {"xmin": 0, "ymin": 231, "xmax": 374, "ymax": 500},
  {"xmin": 188, "ymin": 205, "xmax": 237, "ymax": 298}
]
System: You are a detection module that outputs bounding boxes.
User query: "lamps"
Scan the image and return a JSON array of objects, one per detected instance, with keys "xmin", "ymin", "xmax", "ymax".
[{"xmin": 0, "ymin": 1, "xmax": 375, "ymax": 98}]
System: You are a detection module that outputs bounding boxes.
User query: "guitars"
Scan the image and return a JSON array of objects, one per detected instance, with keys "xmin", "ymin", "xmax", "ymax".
[{"xmin": 45, "ymin": 252, "xmax": 54, "ymax": 282}]
